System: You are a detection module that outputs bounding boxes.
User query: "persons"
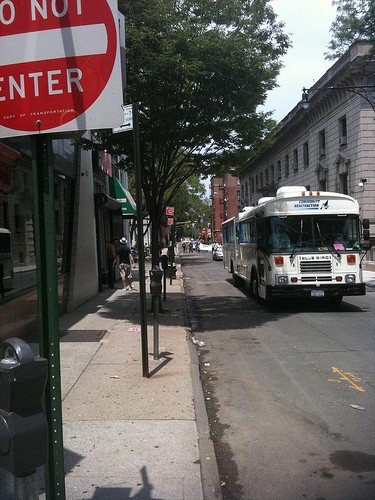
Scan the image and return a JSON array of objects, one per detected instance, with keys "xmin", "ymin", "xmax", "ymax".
[
  {"xmin": 107, "ymin": 238, "xmax": 117, "ymax": 289},
  {"xmin": 188, "ymin": 242, "xmax": 193, "ymax": 253},
  {"xmin": 182, "ymin": 243, "xmax": 186, "ymax": 252},
  {"xmin": 211, "ymin": 242, "xmax": 222, "ymax": 252},
  {"xmin": 186, "ymin": 243, "xmax": 188, "ymax": 253},
  {"xmin": 195, "ymin": 241, "xmax": 200, "ymax": 252},
  {"xmin": 116, "ymin": 237, "xmax": 136, "ymax": 291},
  {"xmin": 268, "ymin": 223, "xmax": 290, "ymax": 249}
]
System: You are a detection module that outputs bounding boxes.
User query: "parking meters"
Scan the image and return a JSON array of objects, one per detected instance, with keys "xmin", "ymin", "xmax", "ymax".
[
  {"xmin": 0, "ymin": 338, "xmax": 50, "ymax": 499},
  {"xmin": 159, "ymin": 255, "xmax": 169, "ymax": 302},
  {"xmin": 148, "ymin": 265, "xmax": 164, "ymax": 360}
]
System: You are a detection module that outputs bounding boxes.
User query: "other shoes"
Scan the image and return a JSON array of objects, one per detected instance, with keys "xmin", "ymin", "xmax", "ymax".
[
  {"xmin": 122, "ymin": 289, "xmax": 127, "ymax": 291},
  {"xmin": 129, "ymin": 287, "xmax": 131, "ymax": 291}
]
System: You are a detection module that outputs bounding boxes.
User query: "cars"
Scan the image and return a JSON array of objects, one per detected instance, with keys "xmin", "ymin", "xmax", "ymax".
[{"xmin": 181, "ymin": 240, "xmax": 224, "ymax": 261}]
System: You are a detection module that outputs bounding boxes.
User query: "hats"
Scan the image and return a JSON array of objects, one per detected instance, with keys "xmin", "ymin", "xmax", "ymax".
[{"xmin": 119, "ymin": 237, "xmax": 127, "ymax": 244}]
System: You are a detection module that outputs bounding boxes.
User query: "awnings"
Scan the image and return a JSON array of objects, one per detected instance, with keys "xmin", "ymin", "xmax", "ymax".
[{"xmin": 110, "ymin": 176, "xmax": 138, "ymax": 215}]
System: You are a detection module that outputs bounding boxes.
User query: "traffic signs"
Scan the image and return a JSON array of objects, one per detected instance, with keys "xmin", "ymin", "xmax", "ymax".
[{"xmin": 112, "ymin": 104, "xmax": 134, "ymax": 134}]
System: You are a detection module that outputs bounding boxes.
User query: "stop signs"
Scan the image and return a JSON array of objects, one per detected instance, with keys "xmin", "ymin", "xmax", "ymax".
[{"xmin": 0, "ymin": 0, "xmax": 118, "ymax": 132}]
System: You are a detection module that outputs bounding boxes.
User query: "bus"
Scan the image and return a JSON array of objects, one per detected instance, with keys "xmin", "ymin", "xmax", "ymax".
[{"xmin": 220, "ymin": 185, "xmax": 375, "ymax": 307}]
